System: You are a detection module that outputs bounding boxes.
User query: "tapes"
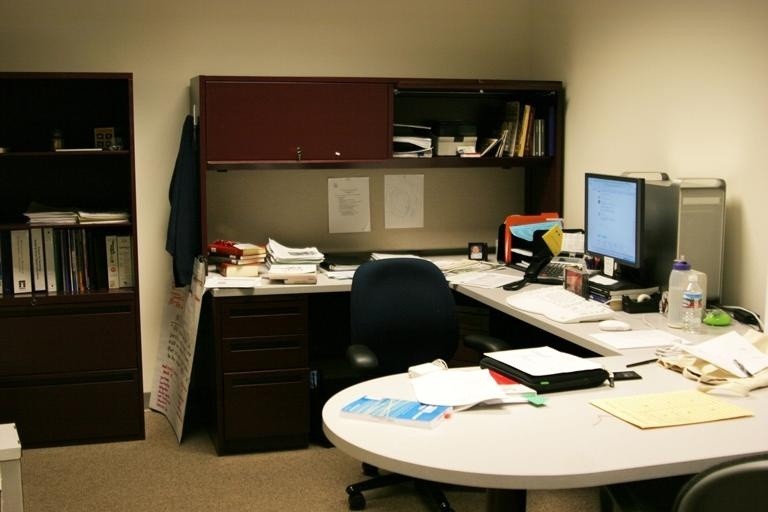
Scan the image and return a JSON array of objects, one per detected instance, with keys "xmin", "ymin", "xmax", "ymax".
[{"xmin": 637, "ymin": 294, "xmax": 651, "ymax": 303}]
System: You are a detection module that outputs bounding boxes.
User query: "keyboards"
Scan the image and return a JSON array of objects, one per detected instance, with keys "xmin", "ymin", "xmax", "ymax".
[{"xmin": 508, "ymin": 286, "xmax": 617, "ymax": 324}]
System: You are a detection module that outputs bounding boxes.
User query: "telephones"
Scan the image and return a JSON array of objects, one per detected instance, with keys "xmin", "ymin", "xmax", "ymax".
[{"xmin": 525, "ymin": 254, "xmax": 585, "ymax": 286}]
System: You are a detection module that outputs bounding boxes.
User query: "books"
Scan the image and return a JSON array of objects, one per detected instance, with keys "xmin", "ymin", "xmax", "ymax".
[
  {"xmin": 11, "ymin": 210, "xmax": 134, "ymax": 294},
  {"xmin": 340, "ymin": 395, "xmax": 452, "ymax": 429},
  {"xmin": 208, "ymin": 238, "xmax": 363, "ymax": 284},
  {"xmin": 393, "ymin": 101, "xmax": 544, "ymax": 157}
]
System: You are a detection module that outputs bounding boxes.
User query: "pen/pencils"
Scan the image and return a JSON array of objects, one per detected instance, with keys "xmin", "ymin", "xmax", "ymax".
[
  {"xmin": 626, "ymin": 359, "xmax": 659, "ymax": 367},
  {"xmin": 735, "ymin": 360, "xmax": 754, "ymax": 377}
]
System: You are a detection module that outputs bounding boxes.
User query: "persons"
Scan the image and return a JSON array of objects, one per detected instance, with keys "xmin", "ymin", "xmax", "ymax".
[{"xmin": 471, "ymin": 246, "xmax": 482, "ymax": 259}]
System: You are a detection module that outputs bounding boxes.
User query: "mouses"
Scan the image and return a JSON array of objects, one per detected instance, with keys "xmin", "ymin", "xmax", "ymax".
[{"xmin": 600, "ymin": 320, "xmax": 631, "ymax": 331}]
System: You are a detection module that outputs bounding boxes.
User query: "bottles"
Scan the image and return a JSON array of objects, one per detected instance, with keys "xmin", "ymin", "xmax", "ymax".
[
  {"xmin": 664, "ymin": 254, "xmax": 692, "ymax": 328},
  {"xmin": 678, "ymin": 276, "xmax": 704, "ymax": 348}
]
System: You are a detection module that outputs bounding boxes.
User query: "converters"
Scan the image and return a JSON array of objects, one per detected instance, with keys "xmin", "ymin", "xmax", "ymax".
[{"xmin": 734, "ymin": 309, "xmax": 759, "ymax": 323}]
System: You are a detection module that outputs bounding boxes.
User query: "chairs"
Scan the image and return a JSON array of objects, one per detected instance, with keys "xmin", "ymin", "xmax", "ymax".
[
  {"xmin": 600, "ymin": 452, "xmax": 768, "ymax": 511},
  {"xmin": 346, "ymin": 258, "xmax": 510, "ymax": 511}
]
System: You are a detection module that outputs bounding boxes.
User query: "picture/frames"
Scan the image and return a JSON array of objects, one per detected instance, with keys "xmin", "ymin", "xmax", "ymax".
[{"xmin": 468, "ymin": 242, "xmax": 488, "ymax": 261}]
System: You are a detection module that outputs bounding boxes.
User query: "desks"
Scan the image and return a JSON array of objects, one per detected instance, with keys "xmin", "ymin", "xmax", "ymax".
[
  {"xmin": 192, "ymin": 250, "xmax": 499, "ymax": 456},
  {"xmin": 321, "ymin": 273, "xmax": 768, "ymax": 512}
]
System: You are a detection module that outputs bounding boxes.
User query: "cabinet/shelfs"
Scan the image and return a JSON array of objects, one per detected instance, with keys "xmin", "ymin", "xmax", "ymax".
[
  {"xmin": 187, "ymin": 73, "xmax": 565, "ymax": 274},
  {"xmin": 0, "ymin": 72, "xmax": 147, "ymax": 449}
]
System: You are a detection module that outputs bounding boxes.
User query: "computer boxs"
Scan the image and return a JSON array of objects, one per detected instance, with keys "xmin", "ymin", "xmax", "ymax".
[{"xmin": 622, "ymin": 172, "xmax": 726, "ymax": 306}]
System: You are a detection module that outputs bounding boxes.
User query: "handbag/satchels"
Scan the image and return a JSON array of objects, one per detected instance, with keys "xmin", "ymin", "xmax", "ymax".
[{"xmin": 655, "ymin": 327, "xmax": 768, "ymax": 397}]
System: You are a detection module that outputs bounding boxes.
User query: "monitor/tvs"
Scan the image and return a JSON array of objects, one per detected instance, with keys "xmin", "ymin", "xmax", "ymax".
[{"xmin": 585, "ymin": 173, "xmax": 645, "ymax": 291}]
